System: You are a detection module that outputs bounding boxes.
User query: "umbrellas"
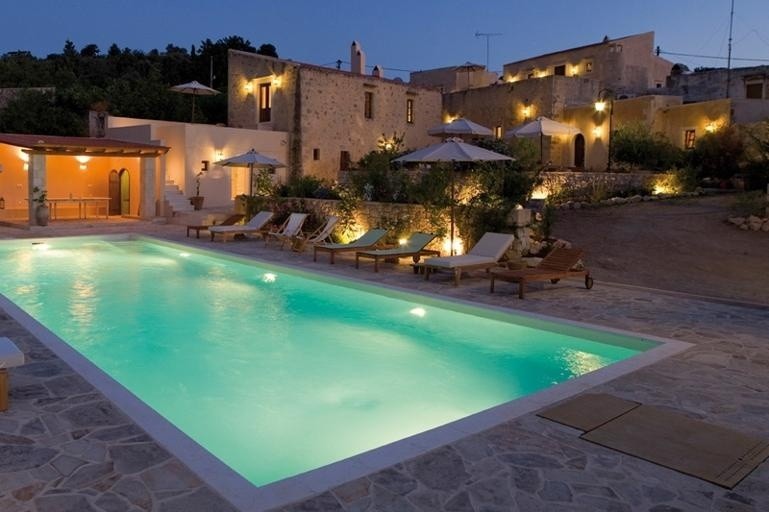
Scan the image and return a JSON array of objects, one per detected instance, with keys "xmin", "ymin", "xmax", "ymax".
[
  {"xmin": 212, "ymin": 147, "xmax": 290, "ymax": 225},
  {"xmin": 426, "ymin": 115, "xmax": 580, "ymax": 161},
  {"xmin": 390, "ymin": 137, "xmax": 517, "ymax": 256},
  {"xmin": 166, "ymin": 79, "xmax": 223, "ymax": 123}
]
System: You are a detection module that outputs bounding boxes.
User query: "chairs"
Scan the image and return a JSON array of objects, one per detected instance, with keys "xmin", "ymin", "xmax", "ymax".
[{"xmin": 187, "ymin": 210, "xmax": 592, "ymax": 299}]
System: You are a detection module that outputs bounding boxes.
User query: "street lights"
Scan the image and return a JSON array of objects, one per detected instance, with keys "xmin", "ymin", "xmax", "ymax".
[{"xmin": 597, "ymin": 88, "xmax": 613, "ymax": 173}]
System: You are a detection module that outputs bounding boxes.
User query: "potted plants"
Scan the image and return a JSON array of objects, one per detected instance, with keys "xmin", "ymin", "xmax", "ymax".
[{"xmin": 32, "ymin": 186, "xmax": 50, "ymax": 226}]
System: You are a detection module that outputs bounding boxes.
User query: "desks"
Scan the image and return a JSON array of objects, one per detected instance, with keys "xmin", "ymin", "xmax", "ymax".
[{"xmin": 43, "ymin": 197, "xmax": 112, "ymax": 221}]
